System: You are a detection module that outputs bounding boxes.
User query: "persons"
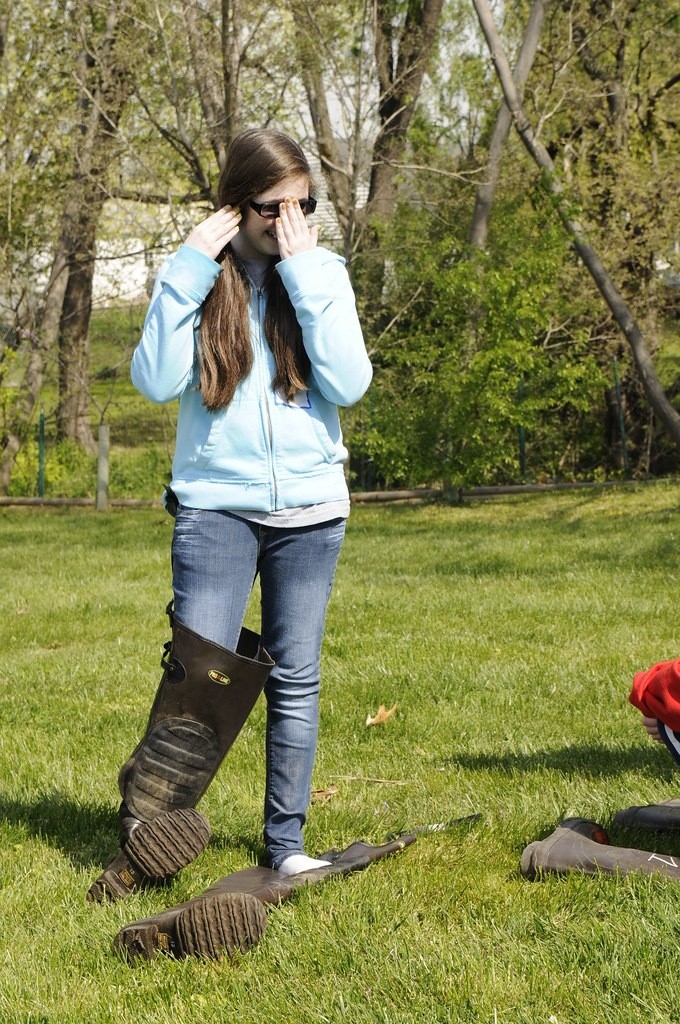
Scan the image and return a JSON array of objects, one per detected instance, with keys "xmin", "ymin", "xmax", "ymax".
[
  {"xmin": 88, "ymin": 128, "xmax": 372, "ymax": 899},
  {"xmin": 629, "ymin": 658, "xmax": 680, "ymax": 764}
]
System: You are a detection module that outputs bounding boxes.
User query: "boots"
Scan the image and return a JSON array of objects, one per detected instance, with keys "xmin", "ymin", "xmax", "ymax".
[
  {"xmin": 110, "ymin": 833, "xmax": 417, "ymax": 967},
  {"xmin": 520, "ymin": 798, "xmax": 680, "ymax": 883},
  {"xmin": 86, "ymin": 599, "xmax": 276, "ymax": 907}
]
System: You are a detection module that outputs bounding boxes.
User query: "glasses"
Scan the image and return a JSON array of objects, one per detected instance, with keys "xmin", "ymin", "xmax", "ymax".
[{"xmin": 249, "ymin": 195, "xmax": 317, "ymax": 220}]
